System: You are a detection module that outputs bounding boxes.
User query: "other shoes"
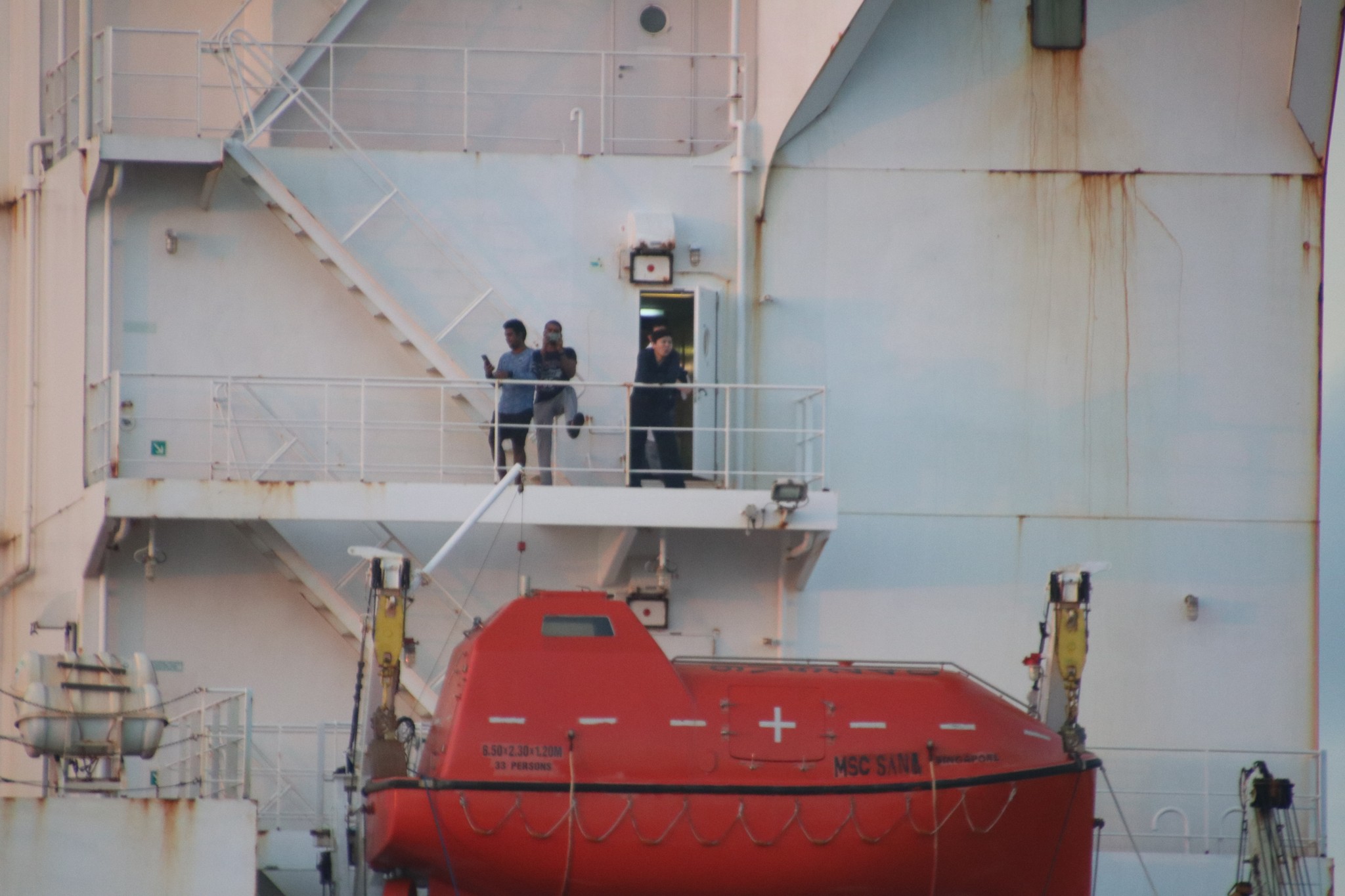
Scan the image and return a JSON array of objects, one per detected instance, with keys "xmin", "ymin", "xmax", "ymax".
[{"xmin": 569, "ymin": 412, "xmax": 585, "ymax": 438}]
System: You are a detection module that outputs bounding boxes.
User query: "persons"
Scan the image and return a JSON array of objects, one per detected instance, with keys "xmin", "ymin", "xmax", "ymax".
[
  {"xmin": 626, "ymin": 319, "xmax": 690, "ymax": 489},
  {"xmin": 530, "ymin": 321, "xmax": 585, "ymax": 488},
  {"xmin": 483, "ymin": 319, "xmax": 539, "ymax": 486}
]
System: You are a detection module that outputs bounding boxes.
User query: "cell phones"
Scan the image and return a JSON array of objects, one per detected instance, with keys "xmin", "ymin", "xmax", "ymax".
[
  {"xmin": 547, "ymin": 333, "xmax": 559, "ymax": 341},
  {"xmin": 481, "ymin": 355, "xmax": 490, "ymax": 365}
]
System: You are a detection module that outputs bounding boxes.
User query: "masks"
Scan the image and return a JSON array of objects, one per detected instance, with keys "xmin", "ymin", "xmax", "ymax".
[{"xmin": 547, "ymin": 332, "xmax": 559, "ymax": 344}]
